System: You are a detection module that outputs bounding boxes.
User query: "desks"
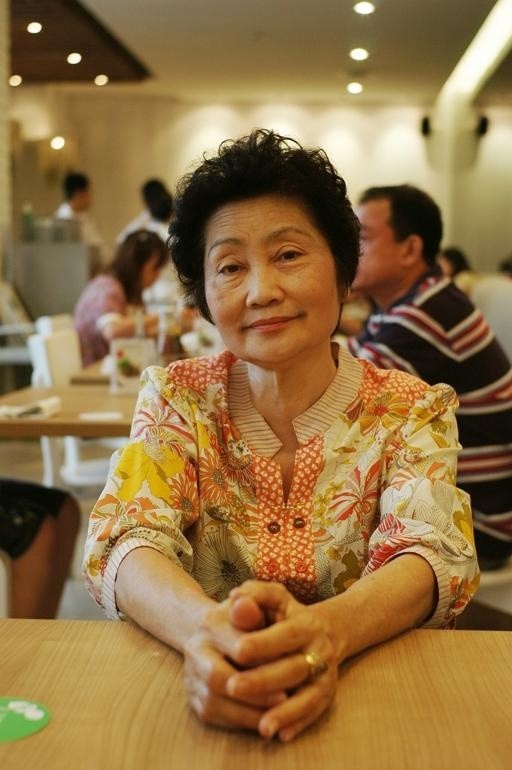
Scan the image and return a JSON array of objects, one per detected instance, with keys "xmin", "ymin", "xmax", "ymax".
[
  {"xmin": 1, "ymin": 314, "xmax": 512, "ymax": 770},
  {"xmin": 1, "ymin": 614, "xmax": 512, "ymax": 770}
]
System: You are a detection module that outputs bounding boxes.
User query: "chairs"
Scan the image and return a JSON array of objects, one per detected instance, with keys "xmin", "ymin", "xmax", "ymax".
[{"xmin": 4, "ymin": 315, "xmax": 226, "ymax": 502}]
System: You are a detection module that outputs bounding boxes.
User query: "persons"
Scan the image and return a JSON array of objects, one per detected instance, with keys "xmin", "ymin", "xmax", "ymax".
[
  {"xmin": 347, "ymin": 186, "xmax": 512, "ymax": 572},
  {"xmin": 467, "ymin": 252, "xmax": 512, "ymax": 369},
  {"xmin": 73, "ymin": 225, "xmax": 201, "ymax": 368},
  {"xmin": 438, "ymin": 248, "xmax": 473, "ymax": 295},
  {"xmin": 114, "ymin": 176, "xmax": 187, "ymax": 306},
  {"xmin": 82, "ymin": 128, "xmax": 483, "ymax": 747},
  {"xmin": 2, "ymin": 474, "xmax": 83, "ymax": 619},
  {"xmin": 337, "ymin": 287, "xmax": 385, "ymax": 336},
  {"xmin": 50, "ymin": 172, "xmax": 109, "ymax": 258}
]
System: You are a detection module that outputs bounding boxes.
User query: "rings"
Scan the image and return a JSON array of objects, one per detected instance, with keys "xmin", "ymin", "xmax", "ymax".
[{"xmin": 304, "ymin": 651, "xmax": 327, "ymax": 679}]
